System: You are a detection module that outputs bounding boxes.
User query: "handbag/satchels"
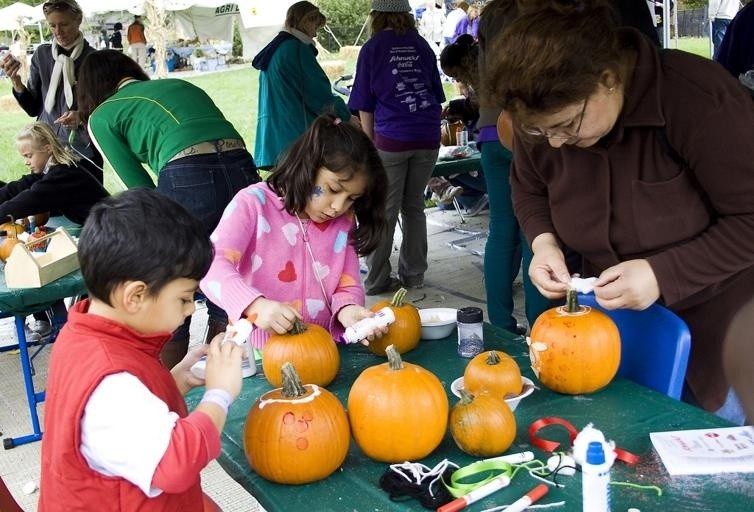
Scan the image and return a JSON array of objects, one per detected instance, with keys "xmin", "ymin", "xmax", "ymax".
[
  {"xmin": 447, "ymin": 98, "xmax": 471, "ymax": 121},
  {"xmin": 441, "ymin": 120, "xmax": 465, "ymax": 146}
]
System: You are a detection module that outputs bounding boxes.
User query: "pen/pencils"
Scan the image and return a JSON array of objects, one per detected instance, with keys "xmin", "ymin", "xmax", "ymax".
[
  {"xmin": 468, "ymin": 451, "xmax": 535, "ymax": 470},
  {"xmin": 502, "ymin": 484, "xmax": 549, "ymax": 512},
  {"xmin": 436, "ymin": 475, "xmax": 512, "ymax": 512}
]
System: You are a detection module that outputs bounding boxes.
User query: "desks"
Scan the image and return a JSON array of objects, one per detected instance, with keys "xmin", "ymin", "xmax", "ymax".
[
  {"xmin": 171, "ymin": 43, "xmax": 229, "ymax": 72},
  {"xmin": 0, "ymin": 214, "xmax": 90, "ymax": 449},
  {"xmin": 185, "ymin": 320, "xmax": 754, "ymax": 512},
  {"xmin": 427, "ymin": 148, "xmax": 484, "ymax": 223}
]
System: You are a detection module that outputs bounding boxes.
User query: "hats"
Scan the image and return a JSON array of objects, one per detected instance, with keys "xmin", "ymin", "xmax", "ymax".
[{"xmin": 373, "ymin": 0, "xmax": 413, "ymax": 13}]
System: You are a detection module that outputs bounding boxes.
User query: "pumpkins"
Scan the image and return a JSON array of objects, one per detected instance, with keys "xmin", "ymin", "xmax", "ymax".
[
  {"xmin": 347, "ymin": 343, "xmax": 449, "ymax": 464},
  {"xmin": 440, "ymin": 121, "xmax": 464, "ymax": 146},
  {"xmin": 529, "ymin": 289, "xmax": 622, "ymax": 395},
  {"xmin": 464, "ymin": 349, "xmax": 523, "ymax": 400},
  {"xmin": 449, "ymin": 388, "xmax": 517, "ymax": 458},
  {"xmin": 497, "ymin": 110, "xmax": 513, "ymax": 152},
  {"xmin": 0, "ymin": 211, "xmax": 51, "ymax": 262},
  {"xmin": 262, "ymin": 318, "xmax": 341, "ymax": 388},
  {"xmin": 242, "ymin": 362, "xmax": 351, "ymax": 485},
  {"xmin": 368, "ymin": 286, "xmax": 422, "ymax": 357}
]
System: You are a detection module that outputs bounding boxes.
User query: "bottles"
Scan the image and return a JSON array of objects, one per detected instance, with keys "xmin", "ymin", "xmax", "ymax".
[
  {"xmin": 191, "ymin": 313, "xmax": 259, "ymax": 381},
  {"xmin": 340, "ymin": 307, "xmax": 395, "ymax": 345},
  {"xmin": 582, "ymin": 443, "xmax": 612, "ymax": 512},
  {"xmin": 457, "ymin": 307, "xmax": 484, "ymax": 358},
  {"xmin": 456, "ymin": 126, "xmax": 468, "ymax": 147}
]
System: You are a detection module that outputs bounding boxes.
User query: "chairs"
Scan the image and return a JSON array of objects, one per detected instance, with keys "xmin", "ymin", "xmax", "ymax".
[{"xmin": 567, "ymin": 288, "xmax": 691, "ymax": 400}]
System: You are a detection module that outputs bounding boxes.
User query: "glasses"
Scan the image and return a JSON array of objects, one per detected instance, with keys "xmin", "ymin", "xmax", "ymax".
[
  {"xmin": 520, "ymin": 96, "xmax": 588, "ymax": 139},
  {"xmin": 44, "ymin": 1, "xmax": 77, "ymax": 10}
]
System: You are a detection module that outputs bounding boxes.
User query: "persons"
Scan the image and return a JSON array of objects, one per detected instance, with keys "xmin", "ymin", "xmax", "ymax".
[
  {"xmin": 346, "ymin": 0, "xmax": 447, "ymax": 296},
  {"xmin": 0, "ymin": 0, "xmax": 103, "ymax": 195},
  {"xmin": 418, "ymin": 0, "xmax": 753, "ymax": 427},
  {"xmin": 100, "ymin": 15, "xmax": 147, "ymax": 72},
  {"xmin": 195, "ymin": 104, "xmax": 389, "ymax": 354},
  {"xmin": 74, "ymin": 48, "xmax": 263, "ymax": 372},
  {"xmin": 0, "ymin": 120, "xmax": 113, "ymax": 342},
  {"xmin": 253, "ymin": 0, "xmax": 361, "ymax": 176},
  {"xmin": 38, "ymin": 187, "xmax": 244, "ymax": 512}
]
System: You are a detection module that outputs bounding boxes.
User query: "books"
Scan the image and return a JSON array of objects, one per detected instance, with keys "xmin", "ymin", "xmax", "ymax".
[{"xmin": 649, "ymin": 425, "xmax": 754, "ymax": 475}]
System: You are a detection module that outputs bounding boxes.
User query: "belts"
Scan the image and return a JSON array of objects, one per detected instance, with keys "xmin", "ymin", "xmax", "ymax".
[{"xmin": 169, "ymin": 136, "xmax": 245, "ymax": 162}]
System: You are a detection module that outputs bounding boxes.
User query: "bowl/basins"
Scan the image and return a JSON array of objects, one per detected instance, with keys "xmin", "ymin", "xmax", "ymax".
[
  {"xmin": 417, "ymin": 306, "xmax": 457, "ymax": 339},
  {"xmin": 451, "ymin": 374, "xmax": 535, "ymax": 413}
]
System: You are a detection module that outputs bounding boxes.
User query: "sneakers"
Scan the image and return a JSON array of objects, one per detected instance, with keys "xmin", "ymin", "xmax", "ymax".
[
  {"xmin": 367, "ymin": 275, "xmax": 423, "ymax": 295},
  {"xmin": 462, "ymin": 193, "xmax": 489, "ymax": 216},
  {"xmin": 439, "ymin": 185, "xmax": 464, "ymax": 205}
]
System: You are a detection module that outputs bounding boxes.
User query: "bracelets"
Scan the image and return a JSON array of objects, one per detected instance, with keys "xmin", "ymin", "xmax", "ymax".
[{"xmin": 198, "ymin": 388, "xmax": 236, "ymax": 416}]
